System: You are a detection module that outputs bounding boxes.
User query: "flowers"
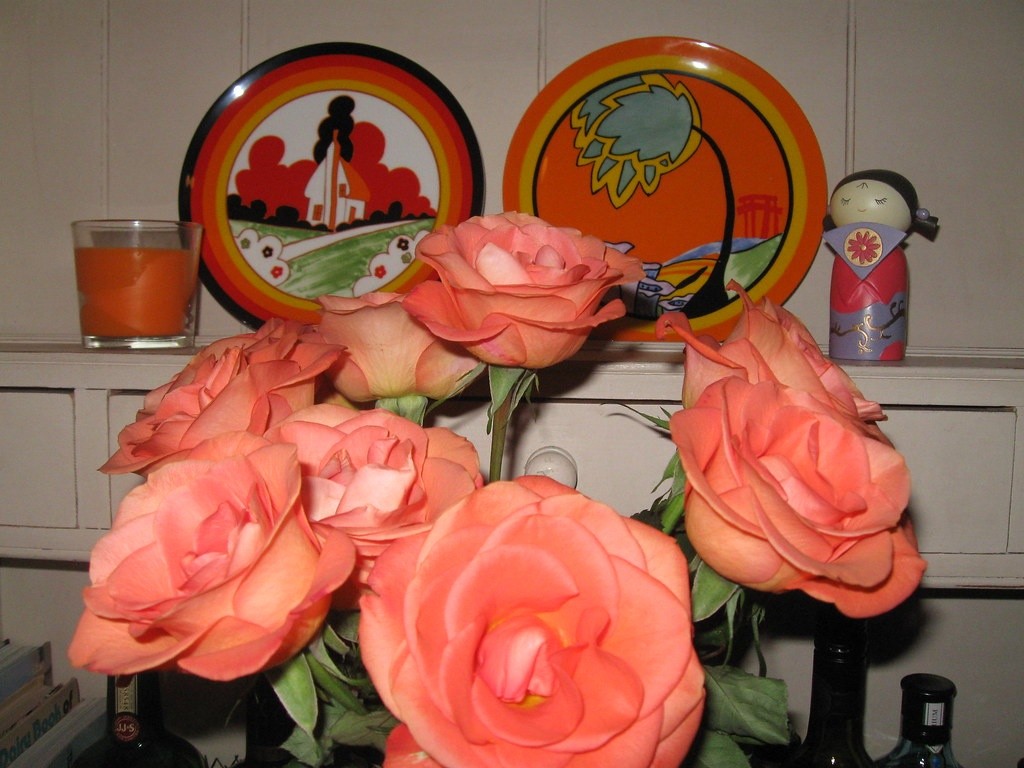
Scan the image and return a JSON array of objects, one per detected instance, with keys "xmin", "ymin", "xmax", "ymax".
[{"xmin": 66, "ymin": 207, "xmax": 933, "ymax": 768}]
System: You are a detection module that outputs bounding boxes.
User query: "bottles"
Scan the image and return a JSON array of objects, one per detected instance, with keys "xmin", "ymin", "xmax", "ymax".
[
  {"xmin": 750, "ymin": 732, "xmax": 800, "ymax": 768},
  {"xmin": 229, "ymin": 673, "xmax": 294, "ymax": 767},
  {"xmin": 791, "ymin": 604, "xmax": 874, "ymax": 768},
  {"xmin": 870, "ymin": 673, "xmax": 962, "ymax": 768},
  {"xmin": 72, "ymin": 669, "xmax": 204, "ymax": 768}
]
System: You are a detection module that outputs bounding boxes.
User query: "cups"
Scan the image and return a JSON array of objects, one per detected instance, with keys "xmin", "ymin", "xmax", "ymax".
[{"xmin": 71, "ymin": 218, "xmax": 202, "ymax": 349}]
